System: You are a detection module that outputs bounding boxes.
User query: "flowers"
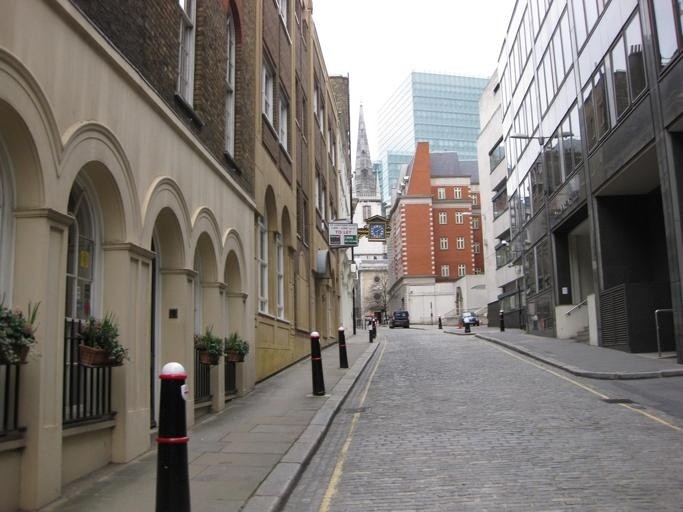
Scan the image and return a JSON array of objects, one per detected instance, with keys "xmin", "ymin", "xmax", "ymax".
[
  {"xmin": 64, "ymin": 311, "xmax": 124, "ymax": 350},
  {"xmin": 0, "ymin": 291, "xmax": 42, "ymax": 364}
]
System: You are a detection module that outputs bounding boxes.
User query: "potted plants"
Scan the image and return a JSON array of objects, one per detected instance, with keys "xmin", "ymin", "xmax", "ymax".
[
  {"xmin": 225, "ymin": 331, "xmax": 250, "ymax": 362},
  {"xmin": 194, "ymin": 322, "xmax": 224, "ymax": 365}
]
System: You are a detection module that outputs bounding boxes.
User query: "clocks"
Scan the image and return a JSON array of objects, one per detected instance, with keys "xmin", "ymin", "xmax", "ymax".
[{"xmin": 368, "ymin": 222, "xmax": 386, "ymax": 241}]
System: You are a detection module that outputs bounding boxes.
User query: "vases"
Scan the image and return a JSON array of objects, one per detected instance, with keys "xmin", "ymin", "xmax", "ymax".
[
  {"xmin": 77, "ymin": 342, "xmax": 109, "ymax": 366},
  {"xmin": 0, "ymin": 343, "xmax": 29, "ymax": 365}
]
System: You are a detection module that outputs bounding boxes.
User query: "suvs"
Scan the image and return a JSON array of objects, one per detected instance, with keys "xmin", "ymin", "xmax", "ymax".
[{"xmin": 387, "ymin": 311, "xmax": 410, "ymax": 328}]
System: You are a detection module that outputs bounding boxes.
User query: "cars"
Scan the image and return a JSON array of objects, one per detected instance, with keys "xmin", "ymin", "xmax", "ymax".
[{"xmin": 458, "ymin": 312, "xmax": 481, "ymax": 327}]
